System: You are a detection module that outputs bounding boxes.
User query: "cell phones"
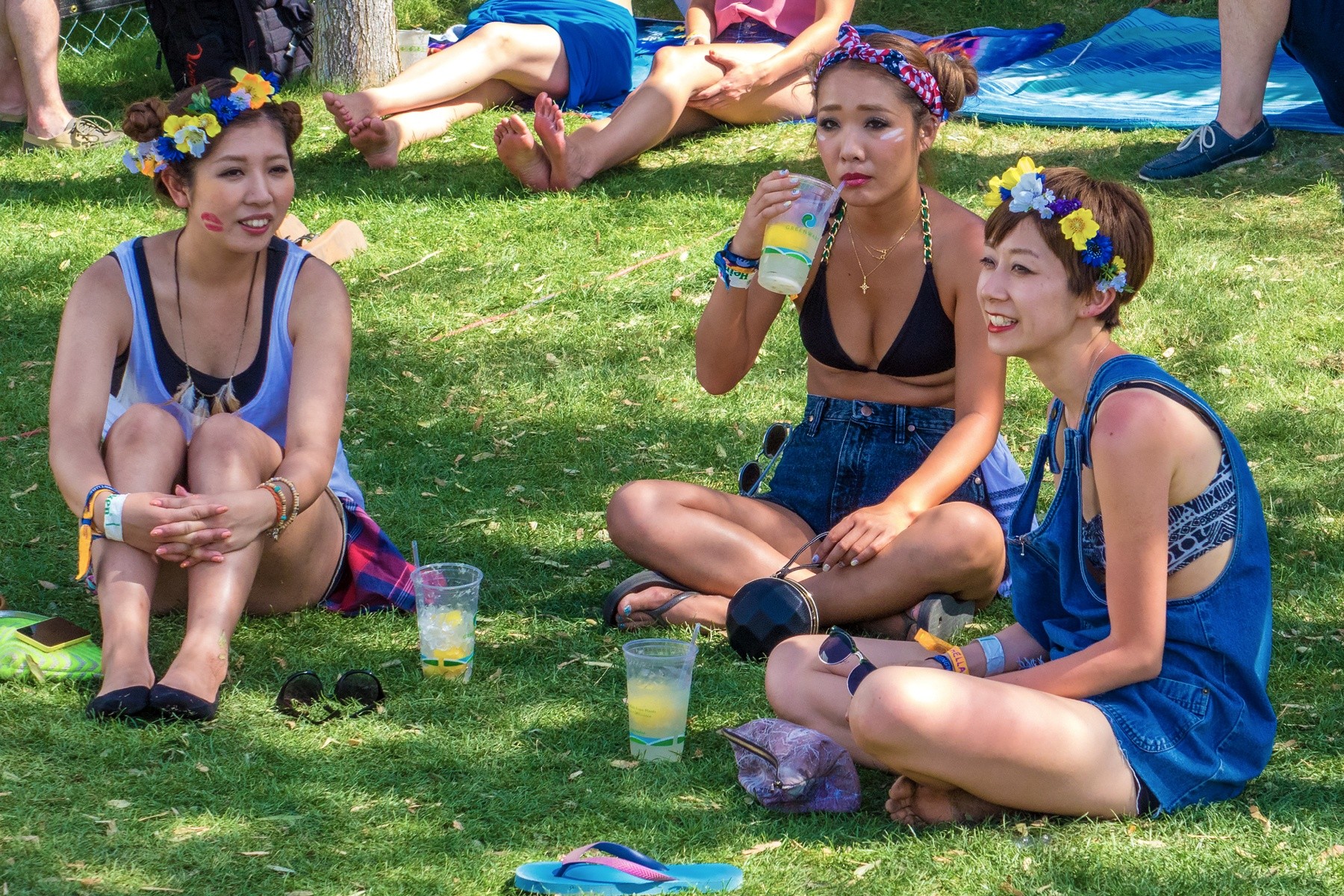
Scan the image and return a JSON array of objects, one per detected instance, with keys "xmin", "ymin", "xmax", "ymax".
[{"xmin": 15, "ymin": 616, "xmax": 92, "ymax": 653}]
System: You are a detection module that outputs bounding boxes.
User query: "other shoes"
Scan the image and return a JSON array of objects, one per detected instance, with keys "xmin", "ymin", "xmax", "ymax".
[
  {"xmin": 1, "ymin": 97, "xmax": 83, "ymax": 132},
  {"xmin": 1137, "ymin": 113, "xmax": 1276, "ymax": 187},
  {"xmin": 20, "ymin": 113, "xmax": 126, "ymax": 151}
]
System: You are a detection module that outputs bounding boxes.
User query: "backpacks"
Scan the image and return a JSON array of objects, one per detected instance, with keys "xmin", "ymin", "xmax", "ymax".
[{"xmin": 145, "ymin": 0, "xmax": 316, "ymax": 96}]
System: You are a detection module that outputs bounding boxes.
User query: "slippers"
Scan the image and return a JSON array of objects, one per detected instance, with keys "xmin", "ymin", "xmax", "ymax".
[
  {"xmin": 601, "ymin": 568, "xmax": 701, "ymax": 634},
  {"xmin": 299, "ymin": 219, "xmax": 367, "ymax": 268},
  {"xmin": 901, "ymin": 592, "xmax": 975, "ymax": 650},
  {"xmin": 515, "ymin": 840, "xmax": 745, "ymax": 896},
  {"xmin": 273, "ymin": 212, "xmax": 312, "ymax": 247}
]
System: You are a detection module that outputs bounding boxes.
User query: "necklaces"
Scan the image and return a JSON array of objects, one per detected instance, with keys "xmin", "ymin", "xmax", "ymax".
[
  {"xmin": 165, "ymin": 230, "xmax": 261, "ymax": 431},
  {"xmin": 841, "ymin": 203, "xmax": 922, "ymax": 295},
  {"xmin": 1064, "ymin": 341, "xmax": 1113, "ymax": 432}
]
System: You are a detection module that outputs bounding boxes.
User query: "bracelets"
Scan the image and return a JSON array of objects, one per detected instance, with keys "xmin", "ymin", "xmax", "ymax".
[
  {"xmin": 256, "ymin": 476, "xmax": 299, "ymax": 543},
  {"xmin": 914, "ymin": 628, "xmax": 968, "ymax": 673},
  {"xmin": 715, "ymin": 235, "xmax": 760, "ymax": 292},
  {"xmin": 76, "ymin": 484, "xmax": 130, "ymax": 581},
  {"xmin": 968, "ymin": 634, "xmax": 1006, "ymax": 678}
]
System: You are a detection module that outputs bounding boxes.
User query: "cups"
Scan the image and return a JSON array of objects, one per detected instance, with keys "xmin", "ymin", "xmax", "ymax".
[
  {"xmin": 622, "ymin": 638, "xmax": 699, "ymax": 763},
  {"xmin": 757, "ymin": 172, "xmax": 840, "ymax": 294},
  {"xmin": 410, "ymin": 562, "xmax": 483, "ymax": 685},
  {"xmin": 395, "ymin": 29, "xmax": 430, "ymax": 71}
]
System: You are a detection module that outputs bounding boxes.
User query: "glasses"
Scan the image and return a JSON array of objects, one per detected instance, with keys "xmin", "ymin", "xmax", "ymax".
[
  {"xmin": 737, "ymin": 421, "xmax": 795, "ymax": 499},
  {"xmin": 274, "ymin": 669, "xmax": 387, "ymax": 727},
  {"xmin": 818, "ymin": 625, "xmax": 879, "ymax": 696}
]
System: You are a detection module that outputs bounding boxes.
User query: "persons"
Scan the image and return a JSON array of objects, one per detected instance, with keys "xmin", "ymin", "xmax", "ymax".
[
  {"xmin": 49, "ymin": 64, "xmax": 445, "ymax": 725},
  {"xmin": 764, "ymin": 158, "xmax": 1283, "ymax": 830},
  {"xmin": 1150, "ymin": 0, "xmax": 1344, "ymax": 180},
  {"xmin": 322, "ymin": 0, "xmax": 636, "ymax": 173},
  {"xmin": 600, "ymin": 24, "xmax": 1035, "ymax": 645},
  {"xmin": 493, "ymin": 0, "xmax": 857, "ymax": 197},
  {"xmin": 0, "ymin": 0, "xmax": 116, "ymax": 151}
]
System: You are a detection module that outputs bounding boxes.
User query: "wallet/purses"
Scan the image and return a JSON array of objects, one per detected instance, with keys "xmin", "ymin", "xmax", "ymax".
[
  {"xmin": 717, "ymin": 718, "xmax": 864, "ymax": 816},
  {"xmin": 726, "ymin": 531, "xmax": 841, "ymax": 664},
  {"xmin": 1, "ymin": 610, "xmax": 104, "ymax": 682}
]
